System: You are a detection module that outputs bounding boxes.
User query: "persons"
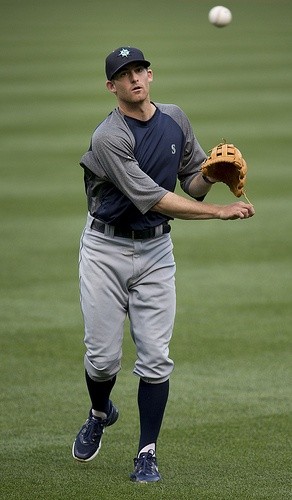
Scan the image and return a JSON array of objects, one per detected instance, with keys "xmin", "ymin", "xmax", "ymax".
[{"xmin": 72, "ymin": 46, "xmax": 255, "ymax": 484}]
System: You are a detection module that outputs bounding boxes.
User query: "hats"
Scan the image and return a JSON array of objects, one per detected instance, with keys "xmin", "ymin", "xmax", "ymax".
[{"xmin": 106, "ymin": 46, "xmax": 150, "ymax": 81}]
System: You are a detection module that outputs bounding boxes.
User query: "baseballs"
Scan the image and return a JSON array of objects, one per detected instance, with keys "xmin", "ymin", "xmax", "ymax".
[{"xmin": 208, "ymin": 6, "xmax": 232, "ymax": 27}]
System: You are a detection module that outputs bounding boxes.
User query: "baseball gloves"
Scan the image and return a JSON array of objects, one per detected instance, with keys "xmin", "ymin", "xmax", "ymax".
[{"xmin": 201, "ymin": 138, "xmax": 253, "ymax": 206}]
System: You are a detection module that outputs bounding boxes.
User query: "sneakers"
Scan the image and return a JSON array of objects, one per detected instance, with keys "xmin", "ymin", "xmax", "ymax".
[
  {"xmin": 130, "ymin": 449, "xmax": 161, "ymax": 484},
  {"xmin": 72, "ymin": 399, "xmax": 119, "ymax": 462}
]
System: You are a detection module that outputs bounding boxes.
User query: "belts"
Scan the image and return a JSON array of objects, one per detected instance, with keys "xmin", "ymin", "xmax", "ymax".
[{"xmin": 90, "ymin": 220, "xmax": 171, "ymax": 240}]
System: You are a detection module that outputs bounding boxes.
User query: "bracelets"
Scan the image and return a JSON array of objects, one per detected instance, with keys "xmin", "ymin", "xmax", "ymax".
[{"xmin": 202, "ymin": 174, "xmax": 216, "ymax": 184}]
